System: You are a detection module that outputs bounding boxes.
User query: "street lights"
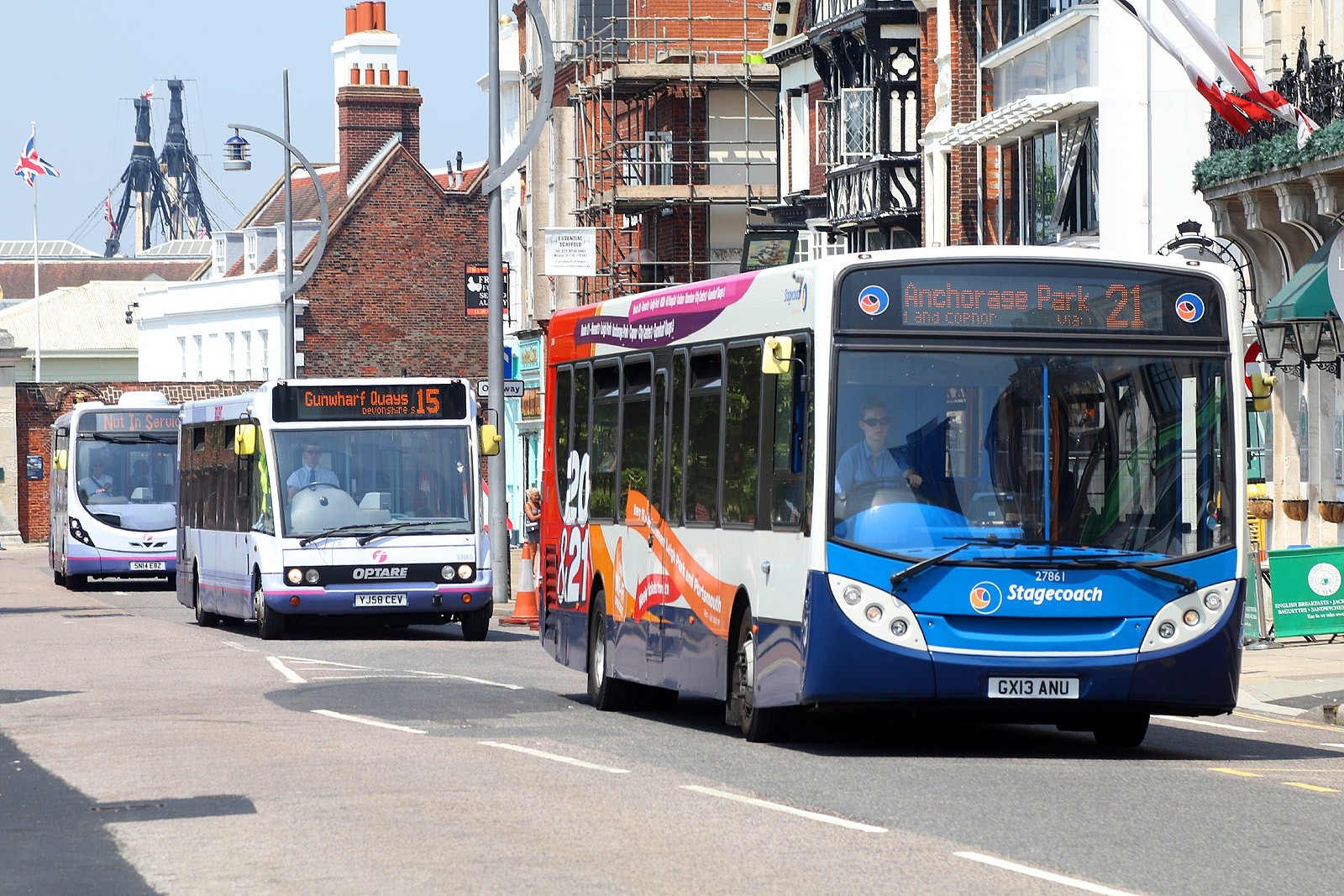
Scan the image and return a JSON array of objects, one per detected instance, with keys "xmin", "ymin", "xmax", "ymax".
[{"xmin": 221, "ymin": 123, "xmax": 331, "ymax": 383}]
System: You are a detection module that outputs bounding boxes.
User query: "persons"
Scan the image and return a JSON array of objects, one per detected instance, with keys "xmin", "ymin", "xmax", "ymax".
[
  {"xmin": 834, "ymin": 397, "xmax": 922, "ymax": 497},
  {"xmin": 78, "ymin": 458, "xmax": 113, "ymax": 499},
  {"xmin": 286, "ymin": 444, "xmax": 340, "ymax": 502},
  {"xmin": 130, "ymin": 460, "xmax": 150, "ymax": 504},
  {"xmin": 525, "ymin": 488, "xmax": 541, "ymax": 576}
]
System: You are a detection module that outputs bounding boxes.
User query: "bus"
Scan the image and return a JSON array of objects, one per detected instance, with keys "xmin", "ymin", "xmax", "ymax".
[
  {"xmin": 536, "ymin": 245, "xmax": 1277, "ymax": 754},
  {"xmin": 175, "ymin": 380, "xmax": 503, "ymax": 641},
  {"xmin": 48, "ymin": 391, "xmax": 182, "ymax": 591}
]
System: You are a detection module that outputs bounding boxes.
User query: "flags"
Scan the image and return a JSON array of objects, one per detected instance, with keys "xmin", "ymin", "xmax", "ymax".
[
  {"xmin": 197, "ymin": 223, "xmax": 208, "ymax": 239},
  {"xmin": 13, "ymin": 132, "xmax": 60, "ymax": 187},
  {"xmin": 141, "ymin": 83, "xmax": 154, "ymax": 98},
  {"xmin": 1164, "ymin": 0, "xmax": 1321, "ymax": 149},
  {"xmin": 105, "ymin": 196, "xmax": 117, "ymax": 233},
  {"xmin": 1113, "ymin": 0, "xmax": 1273, "ymax": 133}
]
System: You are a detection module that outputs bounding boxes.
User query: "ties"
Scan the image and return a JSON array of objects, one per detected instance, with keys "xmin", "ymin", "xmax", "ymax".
[{"xmin": 309, "ymin": 469, "xmax": 316, "ymax": 483}]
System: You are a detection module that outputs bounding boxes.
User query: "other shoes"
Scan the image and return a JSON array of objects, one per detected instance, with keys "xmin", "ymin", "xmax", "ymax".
[{"xmin": 0, "ymin": 547, "xmax": 7, "ymax": 550}]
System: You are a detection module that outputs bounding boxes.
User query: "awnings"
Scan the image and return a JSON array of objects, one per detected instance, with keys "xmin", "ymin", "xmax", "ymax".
[{"xmin": 1266, "ymin": 230, "xmax": 1341, "ymax": 320}]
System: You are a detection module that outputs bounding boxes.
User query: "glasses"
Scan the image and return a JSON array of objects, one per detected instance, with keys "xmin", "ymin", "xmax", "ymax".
[
  {"xmin": 860, "ymin": 415, "xmax": 891, "ymax": 427},
  {"xmin": 303, "ymin": 450, "xmax": 322, "ymax": 455},
  {"xmin": 92, "ymin": 463, "xmax": 104, "ymax": 467}
]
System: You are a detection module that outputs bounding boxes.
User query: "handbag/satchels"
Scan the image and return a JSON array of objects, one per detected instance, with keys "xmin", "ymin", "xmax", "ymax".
[{"xmin": 525, "ymin": 521, "xmax": 540, "ymax": 533}]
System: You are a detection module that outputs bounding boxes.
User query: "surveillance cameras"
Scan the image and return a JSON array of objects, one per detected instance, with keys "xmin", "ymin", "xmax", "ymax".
[{"xmin": 126, "ymin": 311, "xmax": 133, "ymax": 324}]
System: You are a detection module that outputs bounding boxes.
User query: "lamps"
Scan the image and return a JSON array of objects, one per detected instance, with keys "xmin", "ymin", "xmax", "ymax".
[
  {"xmin": 125, "ymin": 303, "xmax": 137, "ymax": 324},
  {"xmin": 1252, "ymin": 311, "xmax": 1342, "ymax": 381}
]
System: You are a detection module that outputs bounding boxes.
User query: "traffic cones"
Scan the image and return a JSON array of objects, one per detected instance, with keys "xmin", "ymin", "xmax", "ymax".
[{"xmin": 499, "ymin": 543, "xmax": 539, "ymax": 629}]
